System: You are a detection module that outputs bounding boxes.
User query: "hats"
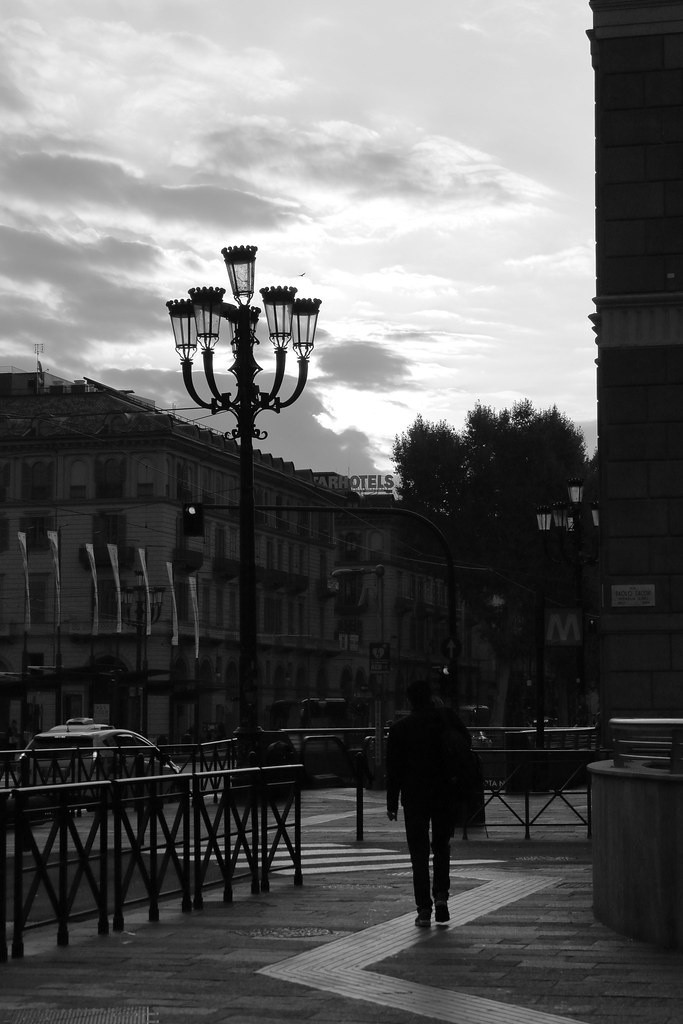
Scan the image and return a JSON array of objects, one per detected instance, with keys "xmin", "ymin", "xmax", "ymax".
[{"xmin": 407, "ymin": 680, "xmax": 432, "ymax": 704}]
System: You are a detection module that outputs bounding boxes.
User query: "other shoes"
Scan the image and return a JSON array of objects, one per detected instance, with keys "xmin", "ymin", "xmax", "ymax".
[
  {"xmin": 415, "ymin": 908, "xmax": 431, "ymax": 925},
  {"xmin": 435, "ymin": 902, "xmax": 449, "ymax": 922}
]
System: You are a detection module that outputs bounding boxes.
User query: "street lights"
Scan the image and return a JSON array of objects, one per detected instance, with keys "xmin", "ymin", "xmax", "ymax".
[
  {"xmin": 167, "ymin": 244, "xmax": 324, "ymax": 790},
  {"xmin": 330, "ymin": 563, "xmax": 388, "ymax": 791},
  {"xmin": 112, "ymin": 570, "xmax": 166, "ymax": 734},
  {"xmin": 534, "ymin": 478, "xmax": 600, "ymax": 727}
]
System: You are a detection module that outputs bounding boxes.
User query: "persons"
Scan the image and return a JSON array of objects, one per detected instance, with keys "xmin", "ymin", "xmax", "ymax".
[{"xmin": 385, "ymin": 678, "xmax": 473, "ymax": 926}]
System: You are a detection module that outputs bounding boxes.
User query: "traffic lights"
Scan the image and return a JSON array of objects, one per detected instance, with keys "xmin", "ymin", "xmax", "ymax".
[{"xmin": 182, "ymin": 501, "xmax": 206, "ymax": 538}]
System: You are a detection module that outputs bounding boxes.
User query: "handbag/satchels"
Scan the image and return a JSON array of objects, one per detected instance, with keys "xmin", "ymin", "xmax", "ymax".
[{"xmin": 439, "ymin": 707, "xmax": 485, "ymax": 840}]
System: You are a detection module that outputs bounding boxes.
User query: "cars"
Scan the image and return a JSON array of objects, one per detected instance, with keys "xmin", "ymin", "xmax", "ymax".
[{"xmin": 18, "ymin": 717, "xmax": 184, "ymax": 800}]
